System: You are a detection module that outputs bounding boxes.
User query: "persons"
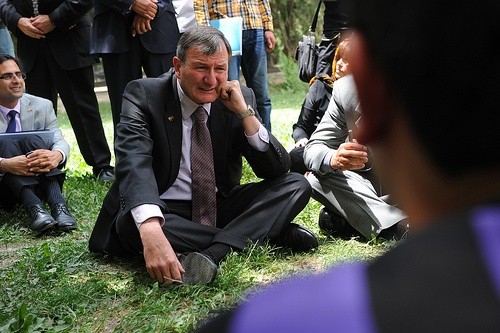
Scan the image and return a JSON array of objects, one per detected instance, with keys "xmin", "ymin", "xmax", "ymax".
[
  {"xmin": 173, "ymin": 0, "xmax": 197, "ymax": 33},
  {"xmin": 191, "ymin": 0, "xmax": 500, "ymax": 333},
  {"xmin": 195, "ymin": 0, "xmax": 277, "ymax": 130},
  {"xmin": 0, "ymin": 0, "xmax": 115, "ymax": 183},
  {"xmin": 90, "ymin": 0, "xmax": 180, "ymax": 141},
  {"xmin": 0, "ymin": 54, "xmax": 77, "ymax": 233},
  {"xmin": 88, "ymin": 25, "xmax": 319, "ymax": 288},
  {"xmin": 289, "ymin": 37, "xmax": 354, "ymax": 174},
  {"xmin": 303, "ymin": 74, "xmax": 411, "ymax": 243},
  {"xmin": 0, "ymin": 20, "xmax": 15, "ymax": 58},
  {"xmin": 316, "ymin": 0, "xmax": 360, "ymax": 76}
]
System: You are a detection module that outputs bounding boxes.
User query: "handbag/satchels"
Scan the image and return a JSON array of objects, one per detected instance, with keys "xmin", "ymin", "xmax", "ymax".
[{"xmin": 295, "ymin": 35, "xmax": 318, "ymax": 83}]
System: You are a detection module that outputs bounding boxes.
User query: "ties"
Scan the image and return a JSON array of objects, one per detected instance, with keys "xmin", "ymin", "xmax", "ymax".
[
  {"xmin": 6, "ymin": 110, "xmax": 18, "ymax": 132},
  {"xmin": 190, "ymin": 107, "xmax": 216, "ymax": 227},
  {"xmin": 32, "ymin": 0, "xmax": 39, "ymax": 17}
]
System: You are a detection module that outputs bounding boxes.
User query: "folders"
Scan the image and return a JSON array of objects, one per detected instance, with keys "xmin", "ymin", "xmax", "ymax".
[{"xmin": 195, "ymin": 16, "xmax": 242, "ymax": 56}]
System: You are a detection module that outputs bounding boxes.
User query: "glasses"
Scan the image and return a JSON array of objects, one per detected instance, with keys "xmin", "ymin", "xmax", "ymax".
[{"xmin": 0, "ymin": 72, "xmax": 26, "ymax": 80}]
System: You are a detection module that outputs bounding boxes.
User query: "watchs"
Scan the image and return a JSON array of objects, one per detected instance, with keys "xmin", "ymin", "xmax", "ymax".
[{"xmin": 237, "ymin": 104, "xmax": 255, "ymax": 120}]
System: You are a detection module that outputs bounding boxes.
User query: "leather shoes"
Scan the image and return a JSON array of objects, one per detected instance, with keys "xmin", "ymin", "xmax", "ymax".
[
  {"xmin": 51, "ymin": 202, "xmax": 76, "ymax": 230},
  {"xmin": 393, "ymin": 219, "xmax": 410, "ymax": 241},
  {"xmin": 28, "ymin": 202, "xmax": 57, "ymax": 232},
  {"xmin": 318, "ymin": 208, "xmax": 357, "ymax": 234},
  {"xmin": 96, "ymin": 169, "xmax": 116, "ymax": 182},
  {"xmin": 271, "ymin": 223, "xmax": 319, "ymax": 252},
  {"xmin": 174, "ymin": 252, "xmax": 217, "ymax": 286}
]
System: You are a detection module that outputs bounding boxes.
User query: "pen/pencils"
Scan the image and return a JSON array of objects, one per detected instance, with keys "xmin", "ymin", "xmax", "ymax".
[{"xmin": 347, "ymin": 130, "xmax": 353, "ymax": 143}]
